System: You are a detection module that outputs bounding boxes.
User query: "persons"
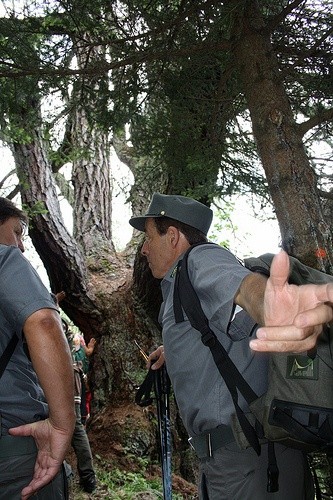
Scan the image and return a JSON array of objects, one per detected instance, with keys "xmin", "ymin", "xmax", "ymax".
[
  {"xmin": 48, "ymin": 292, "xmax": 96, "ymax": 494},
  {"xmin": 127, "ymin": 192, "xmax": 332, "ymax": 500},
  {"xmin": 0, "ymin": 195, "xmax": 77, "ymax": 500}
]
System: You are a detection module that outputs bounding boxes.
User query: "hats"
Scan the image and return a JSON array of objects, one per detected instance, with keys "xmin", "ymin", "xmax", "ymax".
[{"xmin": 129, "ymin": 192, "xmax": 214, "ymax": 237}]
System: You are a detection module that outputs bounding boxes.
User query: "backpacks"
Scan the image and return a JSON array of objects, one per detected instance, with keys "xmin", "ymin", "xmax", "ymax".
[{"xmin": 173, "ymin": 241, "xmax": 333, "ymax": 458}]
